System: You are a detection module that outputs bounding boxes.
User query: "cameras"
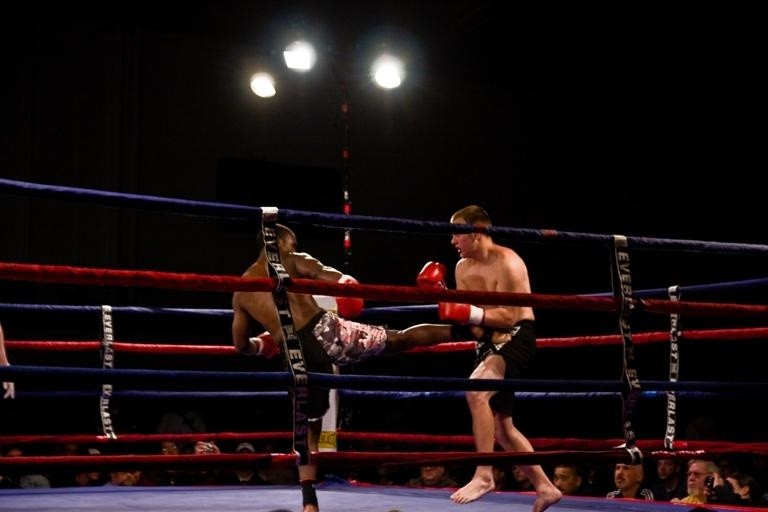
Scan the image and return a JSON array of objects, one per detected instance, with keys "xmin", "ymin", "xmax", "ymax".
[
  {"xmin": 704, "ymin": 475, "xmax": 734, "ymax": 494},
  {"xmin": 201, "ymin": 443, "xmax": 213, "ymax": 452}
]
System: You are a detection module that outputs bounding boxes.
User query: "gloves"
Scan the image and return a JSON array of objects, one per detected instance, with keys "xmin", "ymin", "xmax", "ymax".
[
  {"xmin": 334, "ymin": 275, "xmax": 364, "ymax": 320},
  {"xmin": 438, "ymin": 302, "xmax": 484, "ymax": 327},
  {"xmin": 251, "ymin": 331, "xmax": 281, "ymax": 360},
  {"xmin": 417, "ymin": 260, "xmax": 449, "ymax": 291}
]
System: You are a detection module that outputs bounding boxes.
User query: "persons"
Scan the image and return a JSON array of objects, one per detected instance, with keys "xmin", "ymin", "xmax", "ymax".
[
  {"xmin": 417, "ymin": 205, "xmax": 562, "ymax": 511},
  {"xmin": 1, "ymin": 327, "xmax": 15, "ymax": 400},
  {"xmin": 232, "ymin": 225, "xmax": 511, "ymax": 512},
  {"xmin": 6, "ymin": 426, "xmax": 758, "ymax": 507}
]
